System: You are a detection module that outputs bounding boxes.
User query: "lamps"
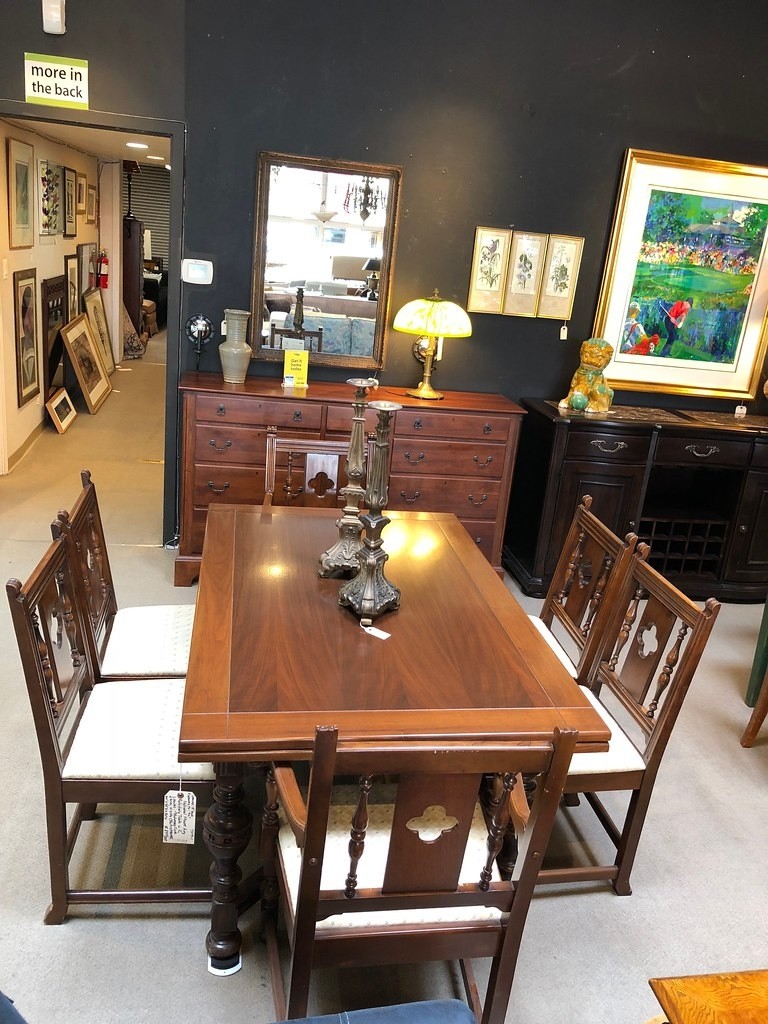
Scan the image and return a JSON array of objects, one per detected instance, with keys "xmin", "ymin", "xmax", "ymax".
[
  {"xmin": 392, "ymin": 288, "xmax": 473, "ymax": 400},
  {"xmin": 311, "ymin": 173, "xmax": 338, "ymax": 224},
  {"xmin": 343, "ymin": 176, "xmax": 387, "ymax": 222},
  {"xmin": 362, "ymin": 257, "xmax": 382, "ymax": 300},
  {"xmin": 122, "ymin": 159, "xmax": 143, "ymax": 220}
]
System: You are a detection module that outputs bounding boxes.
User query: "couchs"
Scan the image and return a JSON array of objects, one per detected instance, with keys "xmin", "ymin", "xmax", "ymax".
[
  {"xmin": 283, "ymin": 314, "xmax": 375, "ymax": 359},
  {"xmin": 143, "ymin": 269, "xmax": 169, "ymax": 330}
]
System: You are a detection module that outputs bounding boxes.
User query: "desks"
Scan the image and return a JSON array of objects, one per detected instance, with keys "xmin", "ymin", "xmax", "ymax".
[
  {"xmin": 176, "ymin": 503, "xmax": 613, "ymax": 973},
  {"xmin": 265, "ymin": 290, "xmax": 378, "ymax": 319}
]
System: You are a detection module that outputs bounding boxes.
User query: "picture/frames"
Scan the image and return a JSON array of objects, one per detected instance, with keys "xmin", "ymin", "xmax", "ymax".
[
  {"xmin": 590, "ymin": 147, "xmax": 768, "ymax": 401},
  {"xmin": 37, "ymin": 158, "xmax": 60, "ymax": 235},
  {"xmin": 64, "ymin": 254, "xmax": 80, "ymax": 324},
  {"xmin": 86, "ymin": 184, "xmax": 96, "ymax": 225},
  {"xmin": 63, "ymin": 166, "xmax": 78, "ymax": 237},
  {"xmin": 13, "ymin": 267, "xmax": 40, "ymax": 408},
  {"xmin": 60, "ymin": 312, "xmax": 113, "ymax": 415},
  {"xmin": 77, "ymin": 172, "xmax": 87, "ymax": 215},
  {"xmin": 78, "ymin": 242, "xmax": 97, "ymax": 314},
  {"xmin": 44, "ymin": 386, "xmax": 78, "ymax": 435},
  {"xmin": 8, "ymin": 136, "xmax": 35, "ymax": 250},
  {"xmin": 43, "ymin": 275, "xmax": 66, "ymax": 407},
  {"xmin": 82, "ymin": 287, "xmax": 116, "ymax": 377},
  {"xmin": 465, "ymin": 226, "xmax": 586, "ymax": 320}
]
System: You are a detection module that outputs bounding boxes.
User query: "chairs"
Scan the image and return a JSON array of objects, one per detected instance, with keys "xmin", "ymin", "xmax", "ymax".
[
  {"xmin": 6, "ymin": 520, "xmax": 219, "ymax": 924},
  {"xmin": 252, "ymin": 725, "xmax": 579, "ymax": 1024},
  {"xmin": 55, "ymin": 470, "xmax": 198, "ymax": 682},
  {"xmin": 265, "ymin": 425, "xmax": 376, "ymax": 510},
  {"xmin": 526, "ymin": 494, "xmax": 639, "ymax": 682},
  {"xmin": 478, "ymin": 541, "xmax": 720, "ymax": 895}
]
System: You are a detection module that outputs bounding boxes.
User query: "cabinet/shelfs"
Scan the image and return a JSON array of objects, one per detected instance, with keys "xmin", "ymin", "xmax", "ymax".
[
  {"xmin": 501, "ymin": 396, "xmax": 768, "ymax": 605},
  {"xmin": 123, "ymin": 220, "xmax": 145, "ymax": 335},
  {"xmin": 175, "ymin": 369, "xmax": 529, "ymax": 588}
]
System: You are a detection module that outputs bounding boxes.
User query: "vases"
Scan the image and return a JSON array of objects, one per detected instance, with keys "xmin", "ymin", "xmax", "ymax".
[{"xmin": 219, "ymin": 308, "xmax": 252, "ymax": 383}]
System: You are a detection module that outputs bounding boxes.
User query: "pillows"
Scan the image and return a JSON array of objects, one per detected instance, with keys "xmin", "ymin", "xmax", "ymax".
[{"xmin": 143, "ymin": 272, "xmax": 163, "ymax": 285}]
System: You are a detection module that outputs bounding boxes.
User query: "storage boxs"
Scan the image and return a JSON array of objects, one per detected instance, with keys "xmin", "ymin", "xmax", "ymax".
[{"xmin": 143, "ymin": 300, "xmax": 158, "ymax": 337}]
